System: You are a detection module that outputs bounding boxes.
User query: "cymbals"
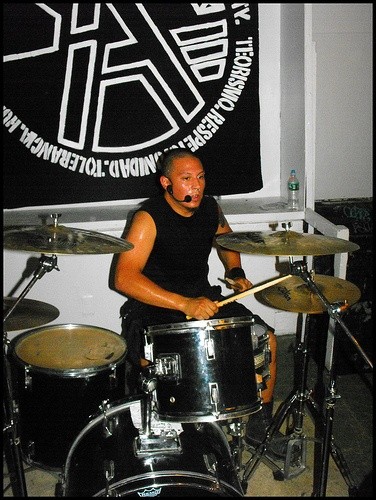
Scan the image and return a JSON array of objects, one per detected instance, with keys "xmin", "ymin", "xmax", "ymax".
[
  {"xmin": 255, "ymin": 272, "xmax": 362, "ymax": 314},
  {"xmin": 215, "ymin": 232, "xmax": 362, "ymax": 256},
  {"xmin": 2, "ymin": 295, "xmax": 60, "ymax": 333},
  {"xmin": 2, "ymin": 224, "xmax": 134, "ymax": 255}
]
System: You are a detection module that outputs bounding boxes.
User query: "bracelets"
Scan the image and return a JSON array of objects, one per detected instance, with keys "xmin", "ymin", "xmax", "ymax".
[{"xmin": 229, "ymin": 267, "xmax": 247, "ymax": 280}]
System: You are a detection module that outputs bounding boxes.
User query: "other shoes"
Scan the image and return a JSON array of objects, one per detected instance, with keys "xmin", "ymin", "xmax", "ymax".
[{"xmin": 244, "ymin": 404, "xmax": 301, "ymax": 462}]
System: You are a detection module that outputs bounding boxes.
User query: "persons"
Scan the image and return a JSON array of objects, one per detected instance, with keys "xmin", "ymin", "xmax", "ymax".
[{"xmin": 114, "ymin": 148, "xmax": 302, "ymax": 465}]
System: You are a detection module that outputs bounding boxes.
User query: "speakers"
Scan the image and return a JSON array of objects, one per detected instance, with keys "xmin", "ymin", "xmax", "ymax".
[{"xmin": 313, "ymin": 197, "xmax": 376, "ymax": 376}]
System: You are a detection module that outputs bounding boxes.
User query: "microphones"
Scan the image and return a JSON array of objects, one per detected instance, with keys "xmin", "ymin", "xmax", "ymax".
[{"xmin": 167, "ymin": 185, "xmax": 192, "ymax": 203}]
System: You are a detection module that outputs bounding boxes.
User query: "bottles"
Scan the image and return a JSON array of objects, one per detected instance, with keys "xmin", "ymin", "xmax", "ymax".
[{"xmin": 287, "ymin": 170, "xmax": 300, "ymax": 211}]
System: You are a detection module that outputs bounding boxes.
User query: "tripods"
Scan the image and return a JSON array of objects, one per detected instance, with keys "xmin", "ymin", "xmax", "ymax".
[{"xmin": 241, "ymin": 313, "xmax": 359, "ymax": 497}]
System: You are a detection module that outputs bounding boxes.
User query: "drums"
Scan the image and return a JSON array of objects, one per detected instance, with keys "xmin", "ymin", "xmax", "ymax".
[
  {"xmin": 69, "ymin": 399, "xmax": 243, "ymax": 498},
  {"xmin": 146, "ymin": 315, "xmax": 271, "ymax": 425},
  {"xmin": 9, "ymin": 322, "xmax": 130, "ymax": 474}
]
